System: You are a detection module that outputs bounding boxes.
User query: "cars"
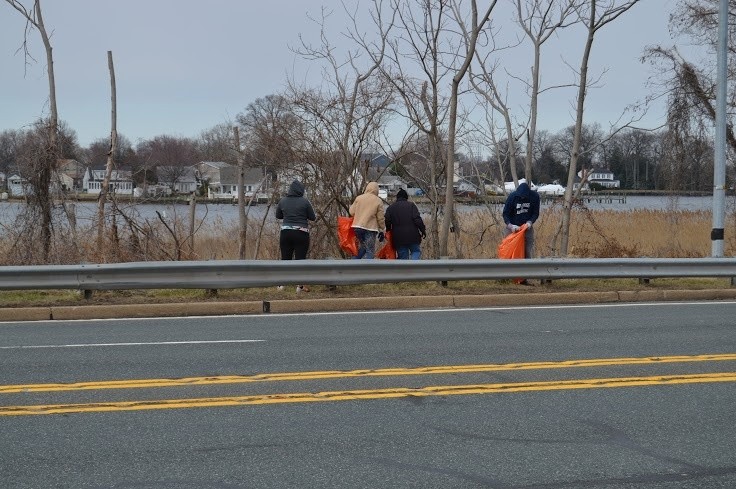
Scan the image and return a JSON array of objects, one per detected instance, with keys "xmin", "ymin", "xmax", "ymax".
[{"xmin": 416, "ymin": 190, "xmax": 427, "ymax": 196}]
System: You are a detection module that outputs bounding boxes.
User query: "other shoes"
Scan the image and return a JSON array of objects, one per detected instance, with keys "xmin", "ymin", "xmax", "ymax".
[
  {"xmin": 297, "ymin": 285, "xmax": 309, "ymax": 292},
  {"xmin": 277, "ymin": 286, "xmax": 285, "ymax": 290}
]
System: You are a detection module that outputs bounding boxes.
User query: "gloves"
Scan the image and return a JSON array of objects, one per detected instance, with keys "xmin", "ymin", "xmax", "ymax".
[
  {"xmin": 377, "ymin": 232, "xmax": 384, "ymax": 242},
  {"xmin": 525, "ymin": 223, "xmax": 531, "ymax": 230},
  {"xmin": 512, "ymin": 225, "xmax": 518, "ymax": 232},
  {"xmin": 420, "ymin": 225, "xmax": 426, "ymax": 239}
]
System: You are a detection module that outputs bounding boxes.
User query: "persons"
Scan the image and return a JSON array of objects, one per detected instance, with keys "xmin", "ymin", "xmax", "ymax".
[
  {"xmin": 275, "ymin": 180, "xmax": 316, "ymax": 293},
  {"xmin": 350, "ymin": 181, "xmax": 385, "ymax": 259},
  {"xmin": 502, "ymin": 178, "xmax": 540, "ymax": 286},
  {"xmin": 386, "ymin": 188, "xmax": 427, "ymax": 260}
]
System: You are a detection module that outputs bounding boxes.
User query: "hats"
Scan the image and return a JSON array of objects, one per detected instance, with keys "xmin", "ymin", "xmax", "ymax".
[{"xmin": 396, "ymin": 187, "xmax": 408, "ymax": 199}]
